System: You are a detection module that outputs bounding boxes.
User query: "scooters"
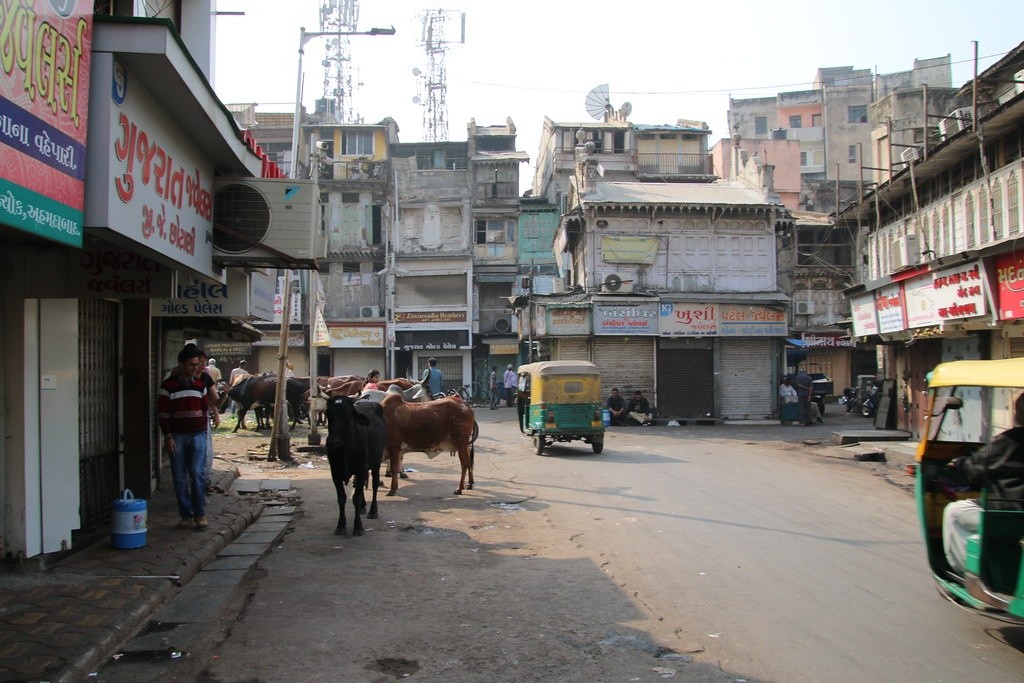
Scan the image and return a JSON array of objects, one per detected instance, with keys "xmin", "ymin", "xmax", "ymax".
[{"xmin": 433, "ymin": 389, "xmax": 479, "ymax": 444}]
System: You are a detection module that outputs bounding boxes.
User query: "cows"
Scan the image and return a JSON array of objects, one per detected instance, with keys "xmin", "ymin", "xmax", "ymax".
[
  {"xmin": 318, "ymin": 389, "xmax": 386, "ymax": 536},
  {"xmin": 226, "ymin": 365, "xmax": 431, "ymax": 434},
  {"xmin": 381, "ymin": 394, "xmax": 476, "ymax": 496}
]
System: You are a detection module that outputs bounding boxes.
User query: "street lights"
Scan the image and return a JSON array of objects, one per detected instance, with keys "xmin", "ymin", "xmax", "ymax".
[{"xmin": 276, "ymin": 27, "xmax": 398, "ymax": 461}]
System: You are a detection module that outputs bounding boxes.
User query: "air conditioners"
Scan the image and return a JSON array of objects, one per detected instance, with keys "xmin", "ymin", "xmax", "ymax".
[
  {"xmin": 212, "ymin": 175, "xmax": 320, "ymax": 262},
  {"xmin": 795, "ymin": 302, "xmax": 815, "ymax": 315},
  {"xmin": 1013, "ymin": 69, "xmax": 1024, "ymax": 94},
  {"xmin": 900, "ymin": 144, "xmax": 924, "ymax": 169},
  {"xmin": 359, "ymin": 306, "xmax": 379, "ymax": 318},
  {"xmin": 602, "ymin": 272, "xmax": 634, "ymax": 294},
  {"xmin": 892, "ymin": 235, "xmax": 920, "ymax": 272},
  {"xmin": 939, "ymin": 105, "xmax": 981, "ymax": 142},
  {"xmin": 553, "ymin": 278, "xmax": 564, "ymax": 292}
]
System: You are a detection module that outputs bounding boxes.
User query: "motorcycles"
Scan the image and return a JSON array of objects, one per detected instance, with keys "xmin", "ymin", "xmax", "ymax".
[
  {"xmin": 517, "ymin": 361, "xmax": 605, "ymax": 455},
  {"xmin": 843, "ymin": 384, "xmax": 881, "ymax": 416},
  {"xmin": 904, "ymin": 358, "xmax": 1024, "ymax": 625}
]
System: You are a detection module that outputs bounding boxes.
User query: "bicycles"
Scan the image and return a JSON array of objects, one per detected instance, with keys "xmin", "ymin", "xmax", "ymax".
[{"xmin": 457, "ymin": 382, "xmax": 490, "ymax": 408}]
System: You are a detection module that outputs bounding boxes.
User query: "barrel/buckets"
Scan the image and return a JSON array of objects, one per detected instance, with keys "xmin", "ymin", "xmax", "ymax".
[
  {"xmin": 109, "ymin": 489, "xmax": 147, "ymax": 549},
  {"xmin": 603, "ymin": 410, "xmax": 610, "ymax": 426}
]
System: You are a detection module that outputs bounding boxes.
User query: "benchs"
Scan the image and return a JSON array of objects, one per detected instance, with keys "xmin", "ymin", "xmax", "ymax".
[{"xmin": 964, "ymin": 492, "xmax": 1024, "ymax": 576}]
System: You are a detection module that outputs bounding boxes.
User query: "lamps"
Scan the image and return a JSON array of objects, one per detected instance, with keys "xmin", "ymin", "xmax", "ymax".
[
  {"xmin": 937, "ymin": 259, "xmax": 944, "ymax": 265},
  {"xmin": 961, "ymin": 252, "xmax": 969, "ymax": 259}
]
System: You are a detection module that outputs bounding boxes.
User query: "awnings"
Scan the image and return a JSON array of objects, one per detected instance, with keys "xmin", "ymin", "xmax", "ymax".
[{"xmin": 787, "ymin": 346, "xmax": 810, "ymax": 367}]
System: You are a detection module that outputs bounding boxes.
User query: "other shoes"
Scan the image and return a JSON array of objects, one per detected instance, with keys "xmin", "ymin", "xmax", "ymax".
[
  {"xmin": 179, "ymin": 517, "xmax": 193, "ymax": 529},
  {"xmin": 193, "ymin": 515, "xmax": 208, "ymax": 527},
  {"xmin": 206, "ymin": 483, "xmax": 214, "ymax": 491}
]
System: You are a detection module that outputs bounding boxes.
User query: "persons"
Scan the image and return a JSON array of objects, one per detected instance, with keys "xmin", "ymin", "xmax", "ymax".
[
  {"xmin": 628, "ymin": 391, "xmax": 652, "ymax": 426},
  {"xmin": 780, "ymin": 379, "xmax": 796, "ymax": 398},
  {"xmin": 504, "ymin": 364, "xmax": 518, "ymax": 406},
  {"xmin": 942, "ymin": 393, "xmax": 1024, "ymax": 577},
  {"xmin": 207, "ymin": 359, "xmax": 222, "ymax": 386},
  {"xmin": 810, "ymin": 401, "xmax": 825, "ymax": 423},
  {"xmin": 160, "ymin": 344, "xmax": 220, "ymax": 529},
  {"xmin": 797, "ymin": 367, "xmax": 813, "ymax": 425},
  {"xmin": 362, "ymin": 369, "xmax": 380, "ymax": 390},
  {"xmin": 607, "ymin": 388, "xmax": 626, "ymax": 426},
  {"xmin": 423, "ymin": 357, "xmax": 443, "ymax": 397},
  {"xmin": 490, "ymin": 365, "xmax": 500, "ymax": 410},
  {"xmin": 229, "ymin": 361, "xmax": 248, "ymax": 419}
]
